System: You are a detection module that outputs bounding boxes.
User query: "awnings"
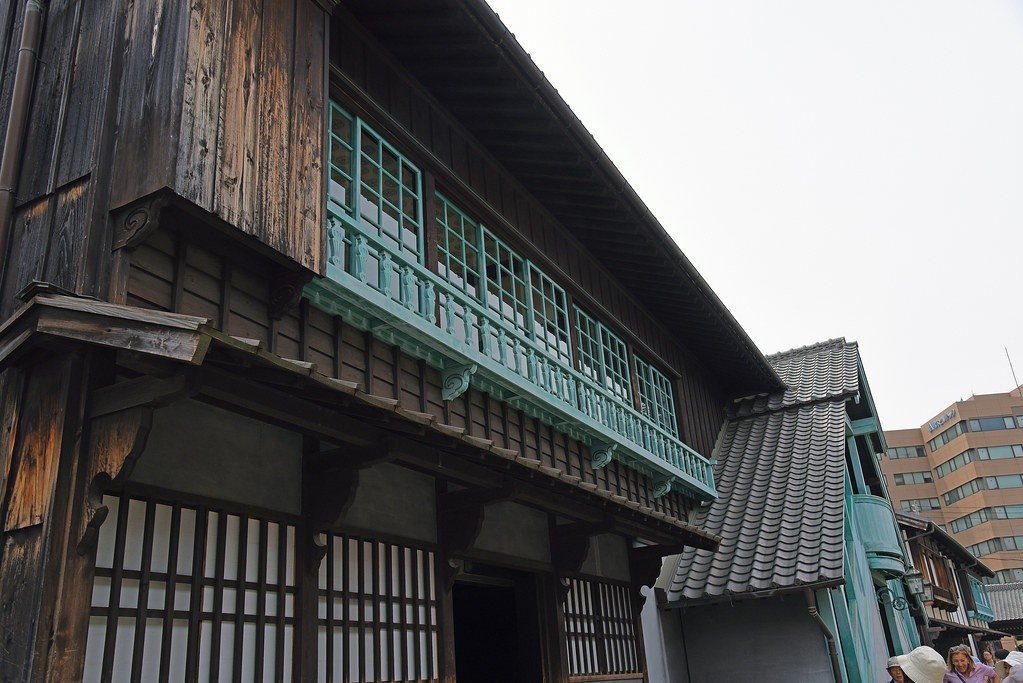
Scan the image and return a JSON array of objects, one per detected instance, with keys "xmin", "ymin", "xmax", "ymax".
[{"xmin": 930, "ymin": 618, "xmax": 1011, "ymax": 641}]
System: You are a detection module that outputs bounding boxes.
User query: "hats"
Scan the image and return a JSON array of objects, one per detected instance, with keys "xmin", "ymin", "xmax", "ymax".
[
  {"xmin": 995, "ymin": 651, "xmax": 1023, "ymax": 679},
  {"xmin": 885, "ymin": 656, "xmax": 900, "ymax": 668},
  {"xmin": 896, "ymin": 645, "xmax": 946, "ymax": 683},
  {"xmin": 995, "ymin": 649, "xmax": 1010, "ymax": 659},
  {"xmin": 1017, "ymin": 644, "xmax": 1023, "ymax": 652}
]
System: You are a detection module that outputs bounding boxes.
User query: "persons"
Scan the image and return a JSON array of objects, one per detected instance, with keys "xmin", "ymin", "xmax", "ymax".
[{"xmin": 886, "ymin": 644, "xmax": 1023, "ymax": 683}]
